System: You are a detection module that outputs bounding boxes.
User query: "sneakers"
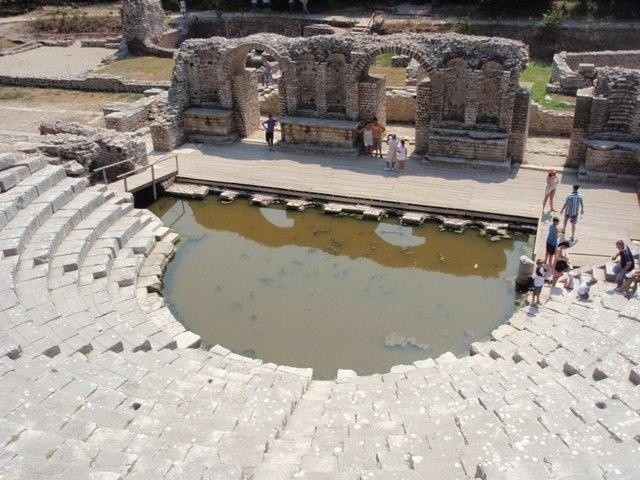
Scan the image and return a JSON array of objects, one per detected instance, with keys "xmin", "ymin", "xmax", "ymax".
[{"xmin": 384, "ymin": 167, "xmax": 405, "ymax": 173}]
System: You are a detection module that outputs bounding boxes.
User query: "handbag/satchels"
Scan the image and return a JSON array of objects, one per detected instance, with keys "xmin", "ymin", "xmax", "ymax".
[{"xmin": 555, "ymin": 260, "xmax": 567, "ymax": 272}]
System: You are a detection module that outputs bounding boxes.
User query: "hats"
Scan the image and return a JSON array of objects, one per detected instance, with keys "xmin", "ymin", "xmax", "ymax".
[
  {"xmin": 549, "ymin": 169, "xmax": 555, "ymax": 173},
  {"xmin": 537, "ymin": 259, "xmax": 544, "ymax": 263}
]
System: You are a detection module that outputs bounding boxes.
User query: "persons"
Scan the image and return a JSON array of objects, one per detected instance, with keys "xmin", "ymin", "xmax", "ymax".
[
  {"xmin": 288, "ymin": 0, "xmax": 310, "ymax": 16},
  {"xmin": 577, "ymin": 277, "xmax": 598, "ymax": 299},
  {"xmin": 386, "ymin": 133, "xmax": 408, "ymax": 173},
  {"xmin": 260, "ymin": 57, "xmax": 271, "ymax": 88},
  {"xmin": 531, "ymin": 217, "xmax": 573, "ymax": 306},
  {"xmin": 262, "ymin": 114, "xmax": 276, "ymax": 151},
  {"xmin": 560, "ymin": 184, "xmax": 585, "ymax": 241},
  {"xmin": 180, "ymin": 0, "xmax": 188, "ymax": 19},
  {"xmin": 250, "ymin": 0, "xmax": 272, "ymax": 14},
  {"xmin": 542, "ymin": 168, "xmax": 560, "ymax": 213},
  {"xmin": 611, "ymin": 240, "xmax": 640, "ymax": 299},
  {"xmin": 355, "ymin": 118, "xmax": 385, "ymax": 158}
]
